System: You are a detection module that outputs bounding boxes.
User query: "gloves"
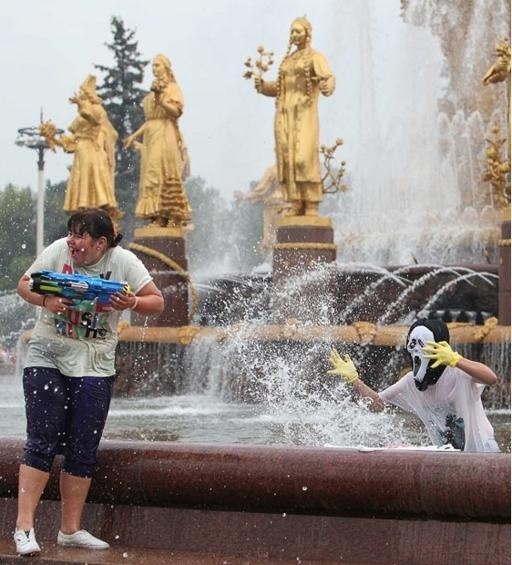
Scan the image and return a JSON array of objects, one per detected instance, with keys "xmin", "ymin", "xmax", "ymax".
[
  {"xmin": 423, "ymin": 340, "xmax": 460, "ymax": 368},
  {"xmin": 327, "ymin": 348, "xmax": 360, "ymax": 383}
]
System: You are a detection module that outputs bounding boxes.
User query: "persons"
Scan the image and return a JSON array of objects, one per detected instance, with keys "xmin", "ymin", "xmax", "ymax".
[
  {"xmin": 327, "ymin": 318, "xmax": 503, "ymax": 452},
  {"xmin": 13, "ymin": 207, "xmax": 165, "ymax": 555},
  {"xmin": 41, "ymin": 75, "xmax": 120, "ymax": 220},
  {"xmin": 254, "ymin": 16, "xmax": 336, "ymax": 217},
  {"xmin": 121, "ymin": 54, "xmax": 191, "ymax": 228}
]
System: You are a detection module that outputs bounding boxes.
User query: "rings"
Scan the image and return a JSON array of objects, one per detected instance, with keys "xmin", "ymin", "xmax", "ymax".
[{"xmin": 116, "ymin": 297, "xmax": 120, "ymax": 302}]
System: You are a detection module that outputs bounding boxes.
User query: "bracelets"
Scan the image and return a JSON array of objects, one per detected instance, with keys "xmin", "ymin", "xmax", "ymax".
[
  {"xmin": 131, "ymin": 298, "xmax": 138, "ymax": 311},
  {"xmin": 42, "ymin": 296, "xmax": 47, "ymax": 307}
]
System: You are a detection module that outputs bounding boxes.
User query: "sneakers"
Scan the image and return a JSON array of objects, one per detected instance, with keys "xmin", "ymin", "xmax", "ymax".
[
  {"xmin": 59, "ymin": 529, "xmax": 110, "ymax": 549},
  {"xmin": 13, "ymin": 530, "xmax": 42, "ymax": 557}
]
